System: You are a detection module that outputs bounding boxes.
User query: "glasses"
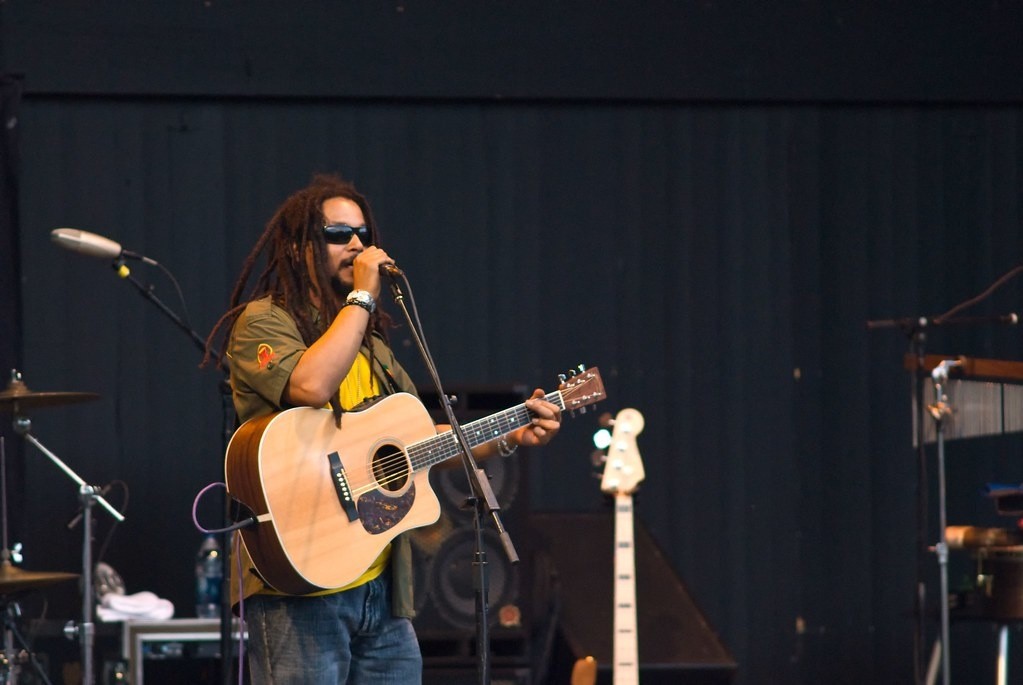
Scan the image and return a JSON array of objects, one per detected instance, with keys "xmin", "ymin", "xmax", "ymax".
[{"xmin": 320, "ymin": 224, "xmax": 373, "ymax": 247}]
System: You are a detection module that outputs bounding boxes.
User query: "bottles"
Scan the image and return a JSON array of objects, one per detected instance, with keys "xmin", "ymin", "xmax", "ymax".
[{"xmin": 195, "ymin": 533, "xmax": 223, "ymax": 619}]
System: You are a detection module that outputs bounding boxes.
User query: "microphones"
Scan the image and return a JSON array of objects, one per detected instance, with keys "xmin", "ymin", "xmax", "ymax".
[
  {"xmin": 50, "ymin": 228, "xmax": 122, "ymax": 259},
  {"xmin": 64, "ymin": 485, "xmax": 113, "ymax": 532},
  {"xmin": 379, "ymin": 263, "xmax": 402, "ymax": 278}
]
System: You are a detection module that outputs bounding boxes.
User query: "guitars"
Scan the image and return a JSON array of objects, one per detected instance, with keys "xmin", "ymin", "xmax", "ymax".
[
  {"xmin": 223, "ymin": 360, "xmax": 612, "ymax": 599},
  {"xmin": 592, "ymin": 406, "xmax": 652, "ymax": 685}
]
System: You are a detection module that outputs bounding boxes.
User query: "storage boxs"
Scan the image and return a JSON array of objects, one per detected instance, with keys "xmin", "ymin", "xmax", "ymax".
[{"xmin": 916, "ymin": 544, "xmax": 1023, "ymax": 623}]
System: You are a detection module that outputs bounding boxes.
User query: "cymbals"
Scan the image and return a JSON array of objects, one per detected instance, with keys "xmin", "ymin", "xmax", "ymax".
[
  {"xmin": 0, "ymin": 563, "xmax": 83, "ymax": 595},
  {"xmin": 0, "ymin": 382, "xmax": 103, "ymax": 415}
]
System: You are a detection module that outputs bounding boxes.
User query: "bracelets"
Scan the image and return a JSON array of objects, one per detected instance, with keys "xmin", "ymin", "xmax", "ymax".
[
  {"xmin": 346, "ymin": 290, "xmax": 375, "ymax": 312},
  {"xmin": 342, "ymin": 301, "xmax": 371, "ymax": 314},
  {"xmin": 496, "ymin": 435, "xmax": 518, "ymax": 456}
]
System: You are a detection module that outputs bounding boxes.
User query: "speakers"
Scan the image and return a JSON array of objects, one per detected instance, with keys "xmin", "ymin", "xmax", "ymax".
[{"xmin": 412, "ymin": 383, "xmax": 538, "ymax": 642}]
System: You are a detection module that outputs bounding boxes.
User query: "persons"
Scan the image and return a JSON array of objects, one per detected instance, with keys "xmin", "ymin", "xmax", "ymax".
[{"xmin": 201, "ymin": 181, "xmax": 561, "ymax": 685}]
